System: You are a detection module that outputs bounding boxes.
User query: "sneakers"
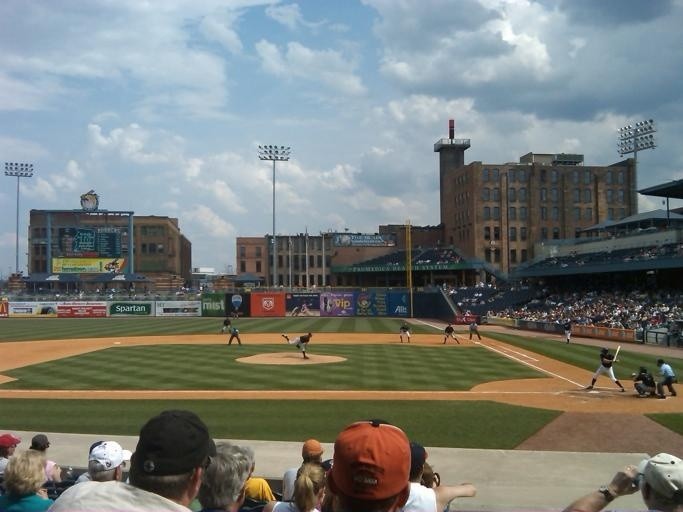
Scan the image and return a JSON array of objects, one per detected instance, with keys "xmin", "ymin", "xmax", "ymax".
[
  {"xmin": 587, "ymin": 385, "xmax": 594, "ymax": 389},
  {"xmin": 281, "ymin": 334, "xmax": 288, "ymax": 337},
  {"xmin": 639, "ymin": 391, "xmax": 676, "ymax": 398},
  {"xmin": 304, "ymin": 355, "xmax": 310, "ymax": 359}
]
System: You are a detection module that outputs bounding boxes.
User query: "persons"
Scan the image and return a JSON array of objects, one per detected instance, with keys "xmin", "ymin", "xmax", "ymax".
[
  {"xmin": 631, "ymin": 366, "xmax": 656, "ymax": 397},
  {"xmin": 657, "ymin": 359, "xmax": 677, "ymax": 399},
  {"xmin": 586, "ymin": 347, "xmax": 625, "ymax": 392}
]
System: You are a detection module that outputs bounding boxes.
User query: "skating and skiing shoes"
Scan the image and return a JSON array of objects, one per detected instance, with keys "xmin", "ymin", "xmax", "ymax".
[{"xmin": 620, "ymin": 386, "xmax": 625, "ymax": 392}]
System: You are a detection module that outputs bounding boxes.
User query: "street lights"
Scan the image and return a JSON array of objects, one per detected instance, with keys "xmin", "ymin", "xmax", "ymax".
[
  {"xmin": 257, "ymin": 144, "xmax": 291, "ymax": 285},
  {"xmin": 617, "ymin": 117, "xmax": 657, "ymax": 217},
  {"xmin": 2, "ymin": 161, "xmax": 34, "ymax": 277}
]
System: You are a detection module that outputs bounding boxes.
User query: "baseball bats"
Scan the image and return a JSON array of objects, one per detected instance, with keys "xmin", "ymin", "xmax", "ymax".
[{"xmin": 613, "ymin": 346, "xmax": 622, "ymax": 361}]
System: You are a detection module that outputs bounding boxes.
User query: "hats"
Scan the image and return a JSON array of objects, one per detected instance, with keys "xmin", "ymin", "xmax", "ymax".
[
  {"xmin": 332, "ymin": 420, "xmax": 412, "ymax": 501},
  {"xmin": 302, "ymin": 439, "xmax": 324, "ymax": 457},
  {"xmin": 638, "ymin": 452, "xmax": 683, "ymax": 501},
  {"xmin": 0, "ymin": 433, "xmax": 21, "ymax": 448},
  {"xmin": 410, "ymin": 442, "xmax": 428, "ymax": 467},
  {"xmin": 89, "ymin": 440, "xmax": 133, "ymax": 471},
  {"xmin": 135, "ymin": 410, "xmax": 217, "ymax": 478},
  {"xmin": 32, "ymin": 434, "xmax": 50, "ymax": 449}
]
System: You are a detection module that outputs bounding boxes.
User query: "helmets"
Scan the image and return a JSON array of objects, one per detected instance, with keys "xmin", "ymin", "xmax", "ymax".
[{"xmin": 600, "ymin": 347, "xmax": 609, "ymax": 353}]
